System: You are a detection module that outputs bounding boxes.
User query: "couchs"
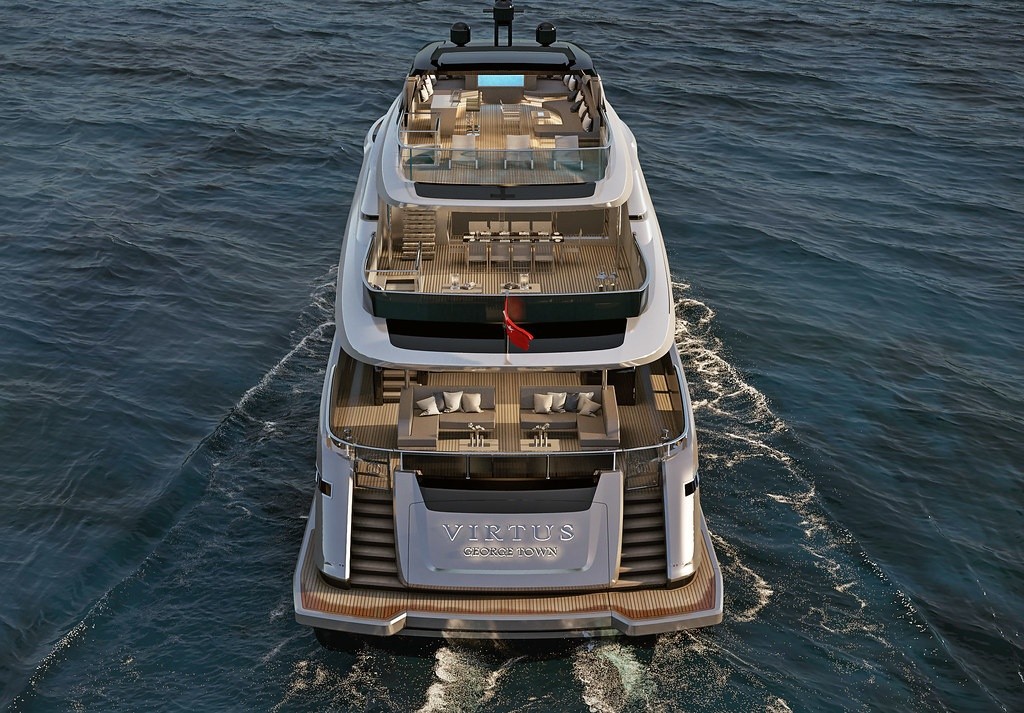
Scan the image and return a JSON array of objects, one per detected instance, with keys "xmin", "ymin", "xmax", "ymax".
[
  {"xmin": 396, "ymin": 385, "xmax": 496, "ymax": 450},
  {"xmin": 533, "ymin": 84, "xmax": 601, "ymax": 149},
  {"xmin": 524, "ymin": 72, "xmax": 583, "ymax": 103},
  {"xmin": 415, "ymin": 74, "xmax": 465, "ymax": 114},
  {"xmin": 519, "ymin": 385, "xmax": 620, "ymax": 451}
]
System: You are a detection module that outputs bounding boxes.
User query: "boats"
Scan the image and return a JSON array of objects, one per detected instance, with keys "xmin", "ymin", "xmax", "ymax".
[{"xmin": 292, "ymin": 0, "xmax": 726, "ymax": 639}]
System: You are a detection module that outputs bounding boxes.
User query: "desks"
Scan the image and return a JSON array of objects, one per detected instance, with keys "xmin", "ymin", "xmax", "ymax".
[
  {"xmin": 442, "ymin": 283, "xmax": 483, "ymax": 294},
  {"xmin": 519, "ymin": 438, "xmax": 560, "ymax": 451},
  {"xmin": 458, "ymin": 439, "xmax": 499, "ymax": 452},
  {"xmin": 499, "ymin": 283, "xmax": 542, "ymax": 294}
]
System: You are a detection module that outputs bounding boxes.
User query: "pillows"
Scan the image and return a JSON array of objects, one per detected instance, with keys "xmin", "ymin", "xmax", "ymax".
[
  {"xmin": 532, "ymin": 393, "xmax": 600, "ymax": 418},
  {"xmin": 420, "ymin": 75, "xmax": 462, "ymax": 103},
  {"xmin": 416, "ymin": 391, "xmax": 484, "ymax": 416},
  {"xmin": 540, "ymin": 75, "xmax": 591, "ymax": 132}
]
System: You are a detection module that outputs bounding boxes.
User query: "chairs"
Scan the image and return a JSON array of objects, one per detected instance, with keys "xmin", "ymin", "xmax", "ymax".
[
  {"xmin": 449, "ymin": 135, "xmax": 479, "ymax": 171},
  {"xmin": 446, "ymin": 221, "xmax": 583, "ymax": 274},
  {"xmin": 552, "ymin": 136, "xmax": 584, "ymax": 171},
  {"xmin": 504, "ymin": 135, "xmax": 533, "ymax": 170},
  {"xmin": 466, "ymin": 92, "xmax": 483, "ymax": 137},
  {"xmin": 499, "ymin": 100, "xmax": 523, "ymax": 134}
]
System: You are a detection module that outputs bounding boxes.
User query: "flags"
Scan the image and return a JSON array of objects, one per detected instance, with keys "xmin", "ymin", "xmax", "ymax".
[{"xmin": 504, "ymin": 297, "xmax": 534, "ymax": 351}]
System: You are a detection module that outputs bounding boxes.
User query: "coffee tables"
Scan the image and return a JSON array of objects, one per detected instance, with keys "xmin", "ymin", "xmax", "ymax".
[{"xmin": 530, "ymin": 111, "xmax": 551, "ymax": 124}]
